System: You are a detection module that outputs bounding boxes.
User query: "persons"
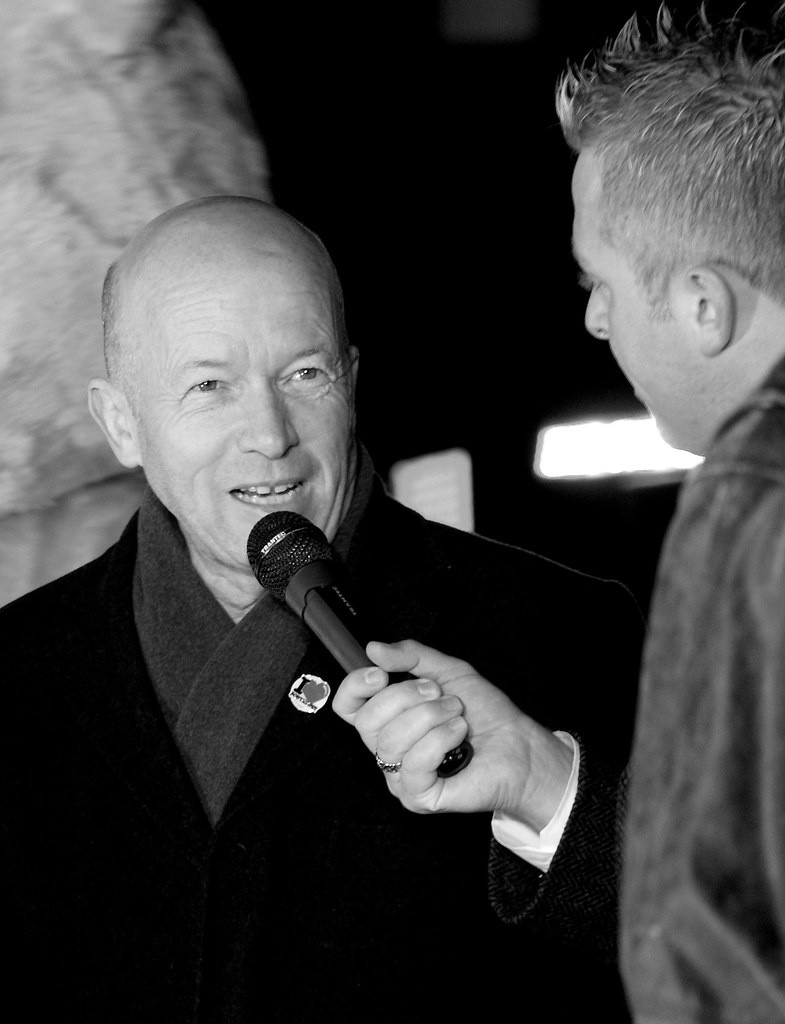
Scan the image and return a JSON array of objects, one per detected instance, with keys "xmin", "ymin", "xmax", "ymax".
[
  {"xmin": 0, "ymin": 195, "xmax": 643, "ymax": 1024},
  {"xmin": 332, "ymin": 0, "xmax": 785, "ymax": 1023}
]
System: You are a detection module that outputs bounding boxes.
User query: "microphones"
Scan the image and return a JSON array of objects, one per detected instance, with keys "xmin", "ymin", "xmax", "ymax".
[{"xmin": 246, "ymin": 511, "xmax": 475, "ymax": 779}]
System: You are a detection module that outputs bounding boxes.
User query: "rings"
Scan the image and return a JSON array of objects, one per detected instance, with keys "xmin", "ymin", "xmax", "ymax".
[{"xmin": 375, "ymin": 749, "xmax": 403, "ymax": 773}]
